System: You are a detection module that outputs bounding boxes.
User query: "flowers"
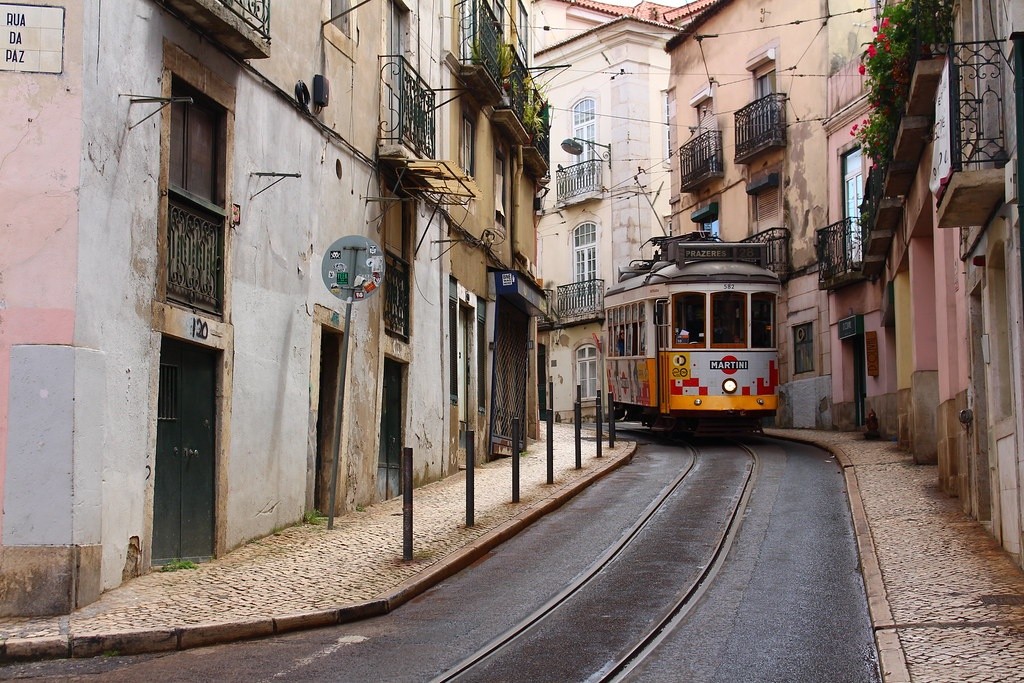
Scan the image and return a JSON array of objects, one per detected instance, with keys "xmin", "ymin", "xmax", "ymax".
[{"xmin": 848, "ymin": 0, "xmax": 954, "ymax": 170}]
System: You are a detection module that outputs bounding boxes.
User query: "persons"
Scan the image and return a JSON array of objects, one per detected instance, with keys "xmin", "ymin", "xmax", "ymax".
[
  {"xmin": 617, "ymin": 328, "xmax": 631, "ymax": 356},
  {"xmin": 698, "ymin": 313, "xmax": 735, "ymax": 344}
]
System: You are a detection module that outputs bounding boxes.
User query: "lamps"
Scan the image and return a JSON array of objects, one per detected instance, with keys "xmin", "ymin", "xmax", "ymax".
[{"xmin": 561, "ymin": 137, "xmax": 611, "ymax": 169}]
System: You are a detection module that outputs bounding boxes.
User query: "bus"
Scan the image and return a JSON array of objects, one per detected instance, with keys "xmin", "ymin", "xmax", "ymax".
[{"xmin": 602, "ymin": 176, "xmax": 780, "ymax": 440}]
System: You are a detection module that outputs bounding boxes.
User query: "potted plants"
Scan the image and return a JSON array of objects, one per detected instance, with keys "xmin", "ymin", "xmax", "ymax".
[{"xmin": 522, "ymin": 103, "xmax": 542, "ymax": 146}]
